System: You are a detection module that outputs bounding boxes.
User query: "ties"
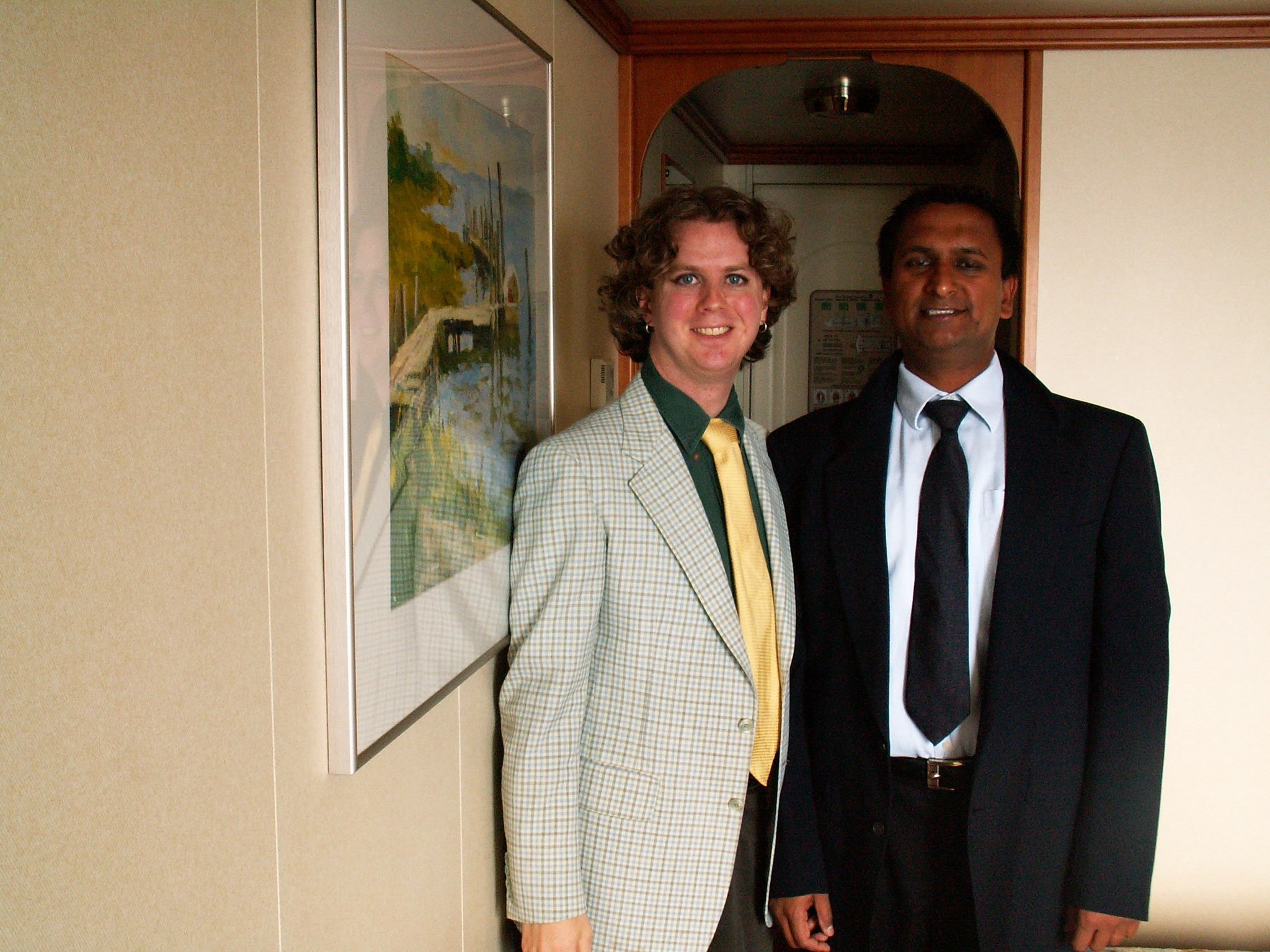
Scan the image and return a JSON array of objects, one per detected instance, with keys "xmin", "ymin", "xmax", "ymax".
[
  {"xmin": 901, "ymin": 400, "xmax": 970, "ymax": 747},
  {"xmin": 702, "ymin": 417, "xmax": 780, "ymax": 788}
]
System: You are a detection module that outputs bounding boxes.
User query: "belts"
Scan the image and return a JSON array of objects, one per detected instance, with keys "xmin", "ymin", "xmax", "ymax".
[{"xmin": 890, "ymin": 757, "xmax": 970, "ymax": 793}]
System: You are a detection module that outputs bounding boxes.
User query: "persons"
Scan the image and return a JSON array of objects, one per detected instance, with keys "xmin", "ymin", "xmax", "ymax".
[
  {"xmin": 498, "ymin": 187, "xmax": 802, "ymax": 952},
  {"xmin": 767, "ymin": 184, "xmax": 1171, "ymax": 952}
]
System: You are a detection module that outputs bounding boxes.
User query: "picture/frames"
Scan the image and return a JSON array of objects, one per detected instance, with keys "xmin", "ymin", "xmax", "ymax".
[{"xmin": 314, "ymin": 0, "xmax": 558, "ymax": 779}]
